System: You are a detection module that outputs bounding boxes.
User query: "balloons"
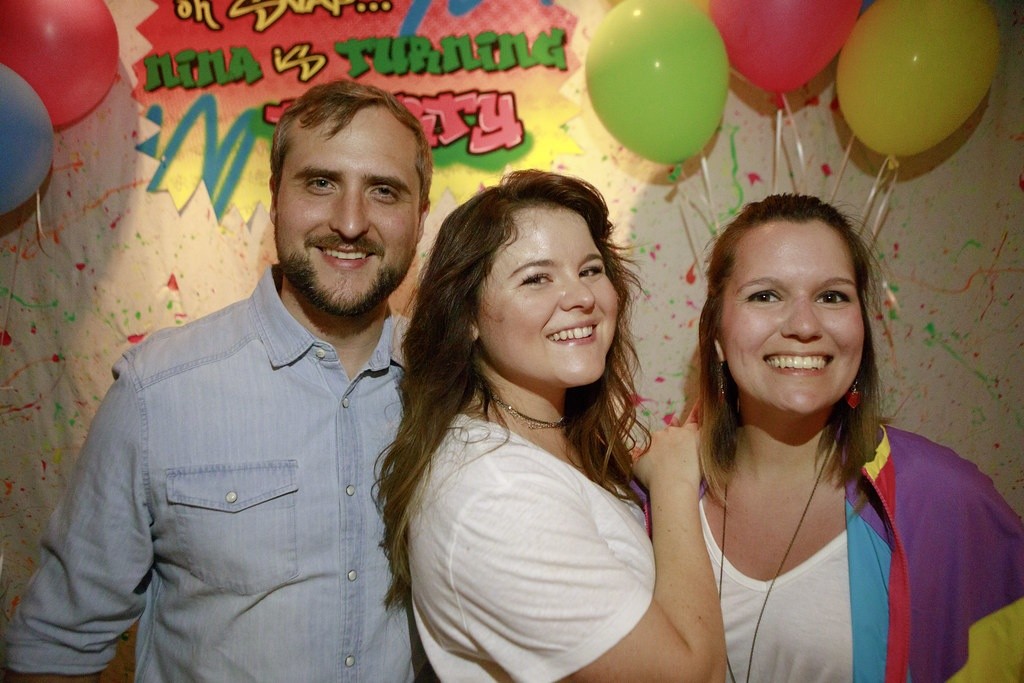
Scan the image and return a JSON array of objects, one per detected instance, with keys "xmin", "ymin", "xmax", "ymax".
[
  {"xmin": 584, "ymin": 0, "xmax": 730, "ymax": 181},
  {"xmin": 0, "ymin": 62, "xmax": 54, "ymax": 216},
  {"xmin": 835, "ymin": 0, "xmax": 1001, "ymax": 169},
  {"xmin": 709, "ymin": 0, "xmax": 862, "ymax": 109},
  {"xmin": 0, "ymin": 0, "xmax": 119, "ymax": 128}
]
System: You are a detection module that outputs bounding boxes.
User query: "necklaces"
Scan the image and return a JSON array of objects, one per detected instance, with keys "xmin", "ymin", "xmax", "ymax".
[
  {"xmin": 487, "ymin": 387, "xmax": 566, "ymax": 430},
  {"xmin": 711, "ymin": 429, "xmax": 833, "ymax": 683}
]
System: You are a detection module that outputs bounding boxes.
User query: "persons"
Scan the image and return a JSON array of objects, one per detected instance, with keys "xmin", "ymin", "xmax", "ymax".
[
  {"xmin": 2, "ymin": 77, "xmax": 433, "ymax": 683},
  {"xmin": 372, "ymin": 168, "xmax": 726, "ymax": 683},
  {"xmin": 631, "ymin": 193, "xmax": 1023, "ymax": 683}
]
